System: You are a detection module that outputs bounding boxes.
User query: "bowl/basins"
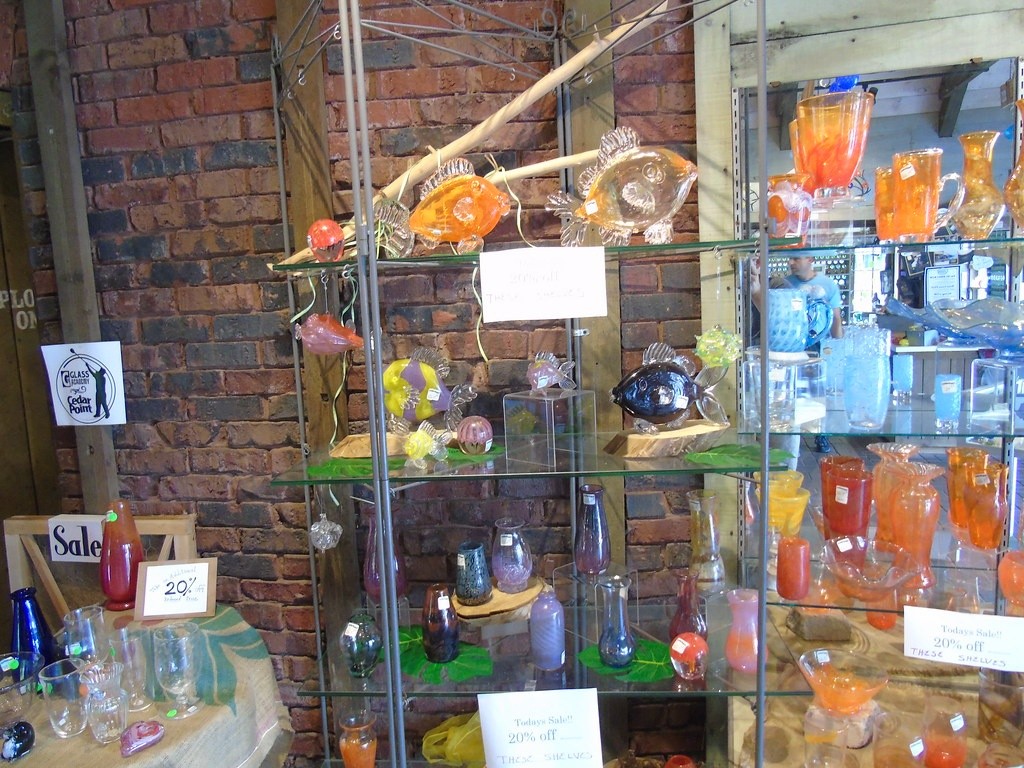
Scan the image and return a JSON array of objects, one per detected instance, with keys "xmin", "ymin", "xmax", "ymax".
[{"xmin": 0, "ymin": 652, "xmax": 45, "ymax": 734}]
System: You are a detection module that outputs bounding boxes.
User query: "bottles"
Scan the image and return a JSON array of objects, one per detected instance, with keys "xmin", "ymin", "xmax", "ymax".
[
  {"xmin": 821, "ymin": 443, "xmax": 989, "ymax": 552},
  {"xmin": 80, "ymin": 662, "xmax": 129, "ymax": 744},
  {"xmin": 949, "ymin": 98, "xmax": 1024, "ymax": 240},
  {"xmin": 767, "ymin": 175, "xmax": 812, "ymax": 250},
  {"xmin": 824, "ymin": 463, "xmax": 1008, "ymax": 589},
  {"xmin": 340, "ymin": 484, "xmax": 768, "ymax": 768}
]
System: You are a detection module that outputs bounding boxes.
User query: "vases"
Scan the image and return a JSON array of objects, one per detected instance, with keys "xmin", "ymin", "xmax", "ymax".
[
  {"xmin": 99, "ymin": 498, "xmax": 146, "ymax": 610},
  {"xmin": 294, "ymin": 91, "xmax": 1024, "ymax": 768},
  {"xmin": 8, "ymin": 587, "xmax": 59, "ymax": 694}
]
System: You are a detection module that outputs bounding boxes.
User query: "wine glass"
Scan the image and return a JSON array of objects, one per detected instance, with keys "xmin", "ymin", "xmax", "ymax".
[
  {"xmin": 789, "ymin": 91, "xmax": 874, "ymax": 209},
  {"xmin": 154, "ymin": 622, "xmax": 205, "ymax": 720},
  {"xmin": 753, "ymin": 471, "xmax": 804, "ymax": 488},
  {"xmin": 756, "ymin": 488, "xmax": 810, "ymax": 576}
]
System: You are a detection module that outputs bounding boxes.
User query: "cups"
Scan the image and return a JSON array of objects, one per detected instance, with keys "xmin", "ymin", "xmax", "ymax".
[
  {"xmin": 63, "ymin": 606, "xmax": 110, "ymax": 669},
  {"xmin": 768, "ymin": 289, "xmax": 834, "ymax": 352},
  {"xmin": 38, "ymin": 658, "xmax": 87, "ymax": 739},
  {"xmin": 803, "ymin": 667, "xmax": 1024, "ymax": 768},
  {"xmin": 820, "ymin": 339, "xmax": 845, "ymax": 401},
  {"xmin": 109, "ymin": 625, "xmax": 155, "ymax": 712},
  {"xmin": 935, "ymin": 374, "xmax": 962, "ymax": 430},
  {"xmin": 893, "ymin": 355, "xmax": 913, "ymax": 397},
  {"xmin": 843, "ymin": 326, "xmax": 891, "ymax": 430},
  {"xmin": 874, "ymin": 148, "xmax": 966, "ymax": 245}
]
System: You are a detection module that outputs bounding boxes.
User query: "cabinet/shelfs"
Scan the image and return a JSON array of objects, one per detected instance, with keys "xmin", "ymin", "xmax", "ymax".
[{"xmin": 267, "ymin": 0, "xmax": 1024, "ymax": 768}]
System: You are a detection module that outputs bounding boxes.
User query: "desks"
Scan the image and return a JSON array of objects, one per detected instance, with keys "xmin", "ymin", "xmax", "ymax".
[{"xmin": 0, "ymin": 602, "xmax": 295, "ymax": 768}]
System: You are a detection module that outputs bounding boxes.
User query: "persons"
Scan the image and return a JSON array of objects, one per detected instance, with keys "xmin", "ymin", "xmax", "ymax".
[
  {"xmin": 784, "ymin": 254, "xmax": 842, "ymax": 454},
  {"xmin": 745, "ymin": 256, "xmax": 795, "ymax": 350}
]
